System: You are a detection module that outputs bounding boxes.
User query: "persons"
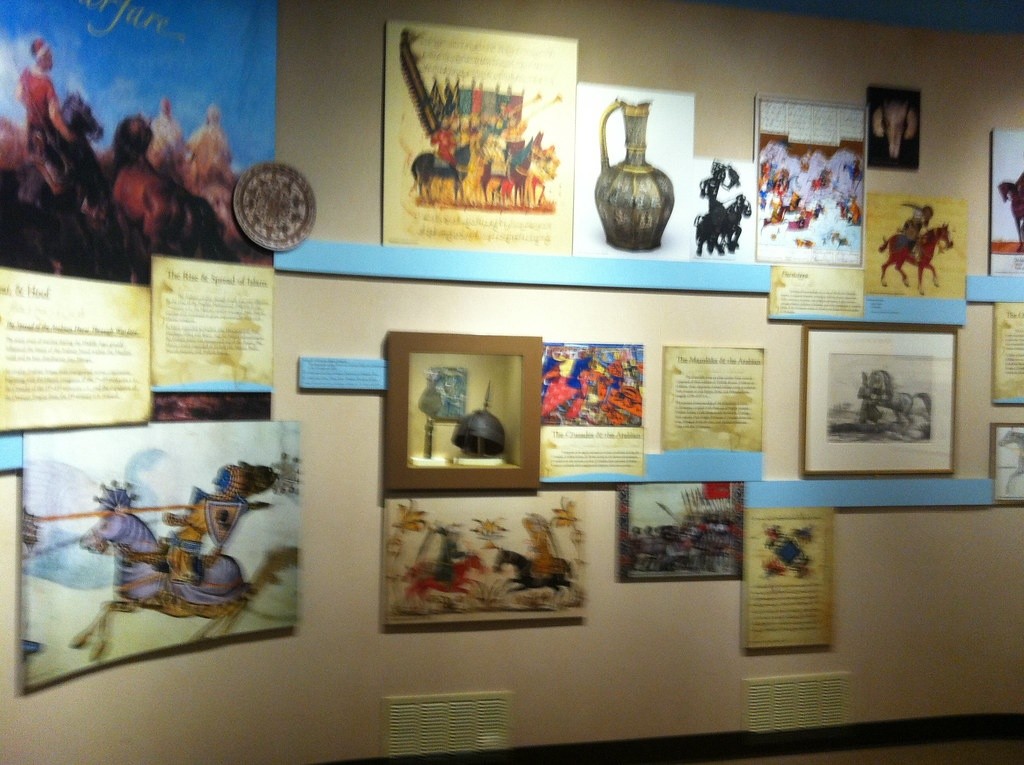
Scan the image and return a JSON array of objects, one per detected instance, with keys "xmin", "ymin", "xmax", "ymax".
[
  {"xmin": 146, "ymin": 94, "xmax": 183, "ymax": 175},
  {"xmin": 188, "ymin": 106, "xmax": 241, "ymax": 241},
  {"xmin": 19, "ymin": 37, "xmax": 81, "ymax": 196}
]
